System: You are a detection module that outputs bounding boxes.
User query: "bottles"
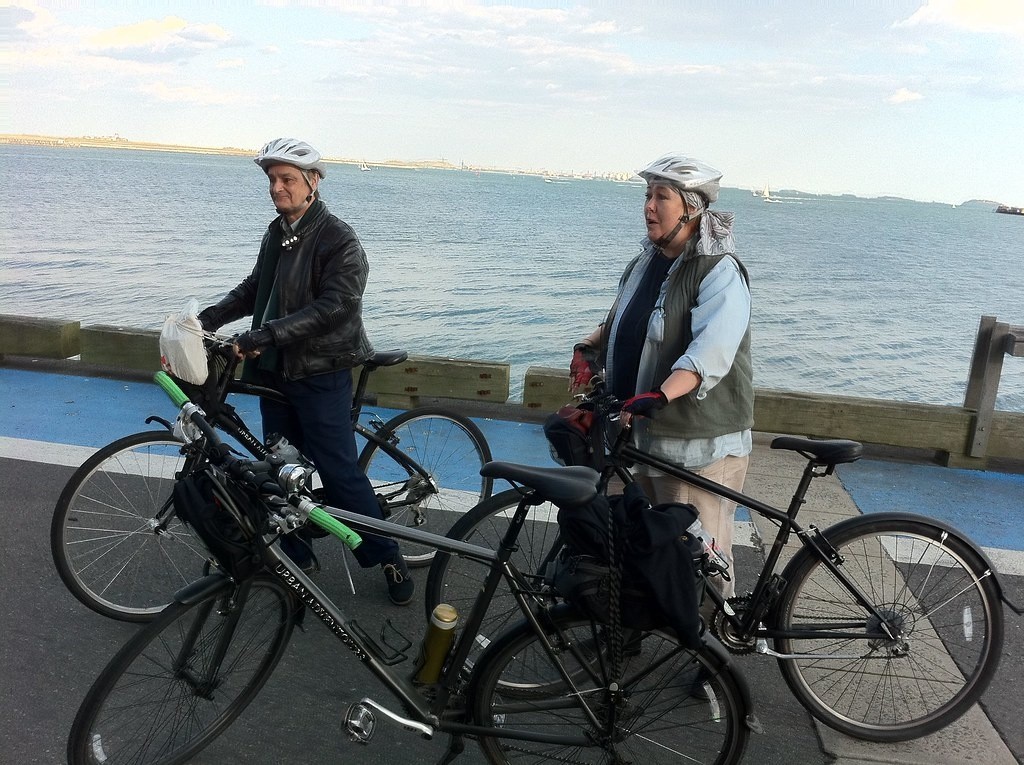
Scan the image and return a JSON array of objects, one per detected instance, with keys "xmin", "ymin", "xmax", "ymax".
[{"xmin": 681, "ymin": 515, "xmax": 728, "ymax": 576}]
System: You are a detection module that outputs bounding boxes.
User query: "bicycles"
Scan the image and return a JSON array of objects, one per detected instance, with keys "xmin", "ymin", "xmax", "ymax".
[
  {"xmin": 66, "ymin": 370, "xmax": 768, "ymax": 765},
  {"xmin": 422, "ymin": 340, "xmax": 1023, "ymax": 745},
  {"xmin": 50, "ymin": 316, "xmax": 497, "ymax": 628}
]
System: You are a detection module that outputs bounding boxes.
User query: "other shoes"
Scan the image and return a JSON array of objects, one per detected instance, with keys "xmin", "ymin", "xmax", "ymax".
[
  {"xmin": 578, "ymin": 624, "xmax": 642, "ymax": 658},
  {"xmin": 691, "ymin": 651, "xmax": 751, "ymax": 701}
]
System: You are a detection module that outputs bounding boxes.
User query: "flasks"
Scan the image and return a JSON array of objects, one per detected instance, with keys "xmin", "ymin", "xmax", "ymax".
[{"xmin": 414, "ymin": 603, "xmax": 459, "ymax": 686}]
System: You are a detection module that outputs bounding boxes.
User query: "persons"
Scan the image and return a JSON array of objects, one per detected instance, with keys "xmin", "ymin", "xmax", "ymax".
[
  {"xmin": 567, "ymin": 153, "xmax": 756, "ymax": 701},
  {"xmin": 191, "ymin": 137, "xmax": 415, "ymax": 608}
]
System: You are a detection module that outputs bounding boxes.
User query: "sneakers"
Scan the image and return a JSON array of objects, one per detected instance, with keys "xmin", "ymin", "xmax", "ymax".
[
  {"xmin": 300, "ymin": 562, "xmax": 321, "ymax": 577},
  {"xmin": 381, "ymin": 551, "xmax": 416, "ymax": 605}
]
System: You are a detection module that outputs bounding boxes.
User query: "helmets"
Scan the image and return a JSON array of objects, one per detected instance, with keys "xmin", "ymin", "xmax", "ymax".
[
  {"xmin": 637, "ymin": 156, "xmax": 724, "ymax": 203},
  {"xmin": 254, "ymin": 138, "xmax": 327, "ymax": 179}
]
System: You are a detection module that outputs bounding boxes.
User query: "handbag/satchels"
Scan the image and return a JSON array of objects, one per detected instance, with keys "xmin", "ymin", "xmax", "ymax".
[
  {"xmin": 553, "ymin": 482, "xmax": 709, "ymax": 649},
  {"xmin": 173, "ymin": 459, "xmax": 270, "ymax": 586},
  {"xmin": 159, "ymin": 299, "xmax": 209, "ymax": 386},
  {"xmin": 543, "ymin": 393, "xmax": 622, "ymax": 472}
]
